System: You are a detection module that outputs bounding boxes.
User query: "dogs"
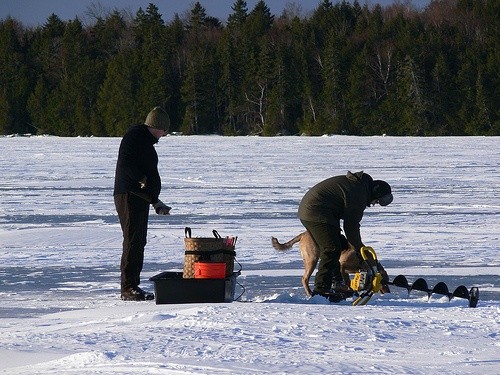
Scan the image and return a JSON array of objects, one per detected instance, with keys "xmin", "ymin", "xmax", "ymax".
[{"xmin": 272, "ymin": 231, "xmax": 391, "ymax": 298}]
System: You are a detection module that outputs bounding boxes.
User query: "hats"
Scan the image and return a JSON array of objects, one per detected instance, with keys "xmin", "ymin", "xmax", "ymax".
[
  {"xmin": 372, "ymin": 177, "xmax": 394, "ymax": 207},
  {"xmin": 144, "ymin": 106, "xmax": 171, "ymax": 131}
]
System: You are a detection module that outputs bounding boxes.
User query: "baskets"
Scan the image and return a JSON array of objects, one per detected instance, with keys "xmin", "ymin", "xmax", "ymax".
[{"xmin": 182, "ymin": 226, "xmax": 238, "ymax": 279}]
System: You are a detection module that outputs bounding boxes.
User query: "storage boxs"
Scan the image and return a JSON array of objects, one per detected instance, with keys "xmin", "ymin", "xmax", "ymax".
[
  {"xmin": 183, "ymin": 226, "xmax": 237, "ymax": 278},
  {"xmin": 150, "ymin": 271, "xmax": 241, "ymax": 305},
  {"xmin": 194, "ymin": 262, "xmax": 226, "ymax": 279}
]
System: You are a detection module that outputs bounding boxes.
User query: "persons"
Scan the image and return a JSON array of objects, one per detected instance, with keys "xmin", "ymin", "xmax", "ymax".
[
  {"xmin": 297, "ymin": 169, "xmax": 393, "ymax": 302},
  {"xmin": 112, "ymin": 105, "xmax": 173, "ymax": 299}
]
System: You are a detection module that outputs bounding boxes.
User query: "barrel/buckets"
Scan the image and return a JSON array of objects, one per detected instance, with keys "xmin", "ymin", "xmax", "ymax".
[{"xmin": 194, "ymin": 262, "xmax": 226, "ymax": 278}]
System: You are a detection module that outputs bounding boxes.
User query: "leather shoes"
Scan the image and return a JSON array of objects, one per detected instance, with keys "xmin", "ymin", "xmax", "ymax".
[{"xmin": 122, "ymin": 287, "xmax": 154, "ymax": 301}]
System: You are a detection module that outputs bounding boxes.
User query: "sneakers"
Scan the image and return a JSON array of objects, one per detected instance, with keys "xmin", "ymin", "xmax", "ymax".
[{"xmin": 312, "ymin": 287, "xmax": 355, "ymax": 303}]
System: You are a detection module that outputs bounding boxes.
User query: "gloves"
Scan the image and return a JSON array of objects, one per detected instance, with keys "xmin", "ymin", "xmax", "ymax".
[{"xmin": 153, "ymin": 199, "xmax": 173, "ymax": 216}]
